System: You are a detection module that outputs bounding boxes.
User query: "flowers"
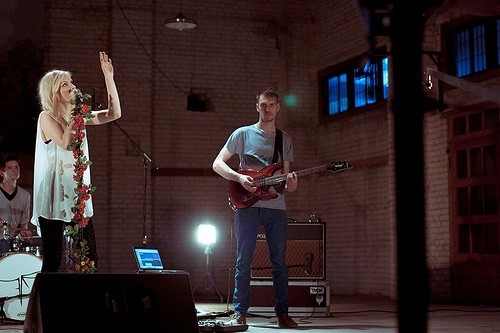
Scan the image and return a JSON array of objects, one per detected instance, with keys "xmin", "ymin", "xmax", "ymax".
[{"xmin": 64, "ymin": 90, "xmax": 98, "ymax": 273}]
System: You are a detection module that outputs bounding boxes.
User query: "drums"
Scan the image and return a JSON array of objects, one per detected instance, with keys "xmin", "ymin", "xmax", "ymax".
[{"xmin": 0, "ymin": 251, "xmax": 43, "ymax": 321}]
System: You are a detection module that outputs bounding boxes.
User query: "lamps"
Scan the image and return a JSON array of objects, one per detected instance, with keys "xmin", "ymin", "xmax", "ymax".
[{"xmin": 162, "ymin": 0, "xmax": 198, "ymax": 31}]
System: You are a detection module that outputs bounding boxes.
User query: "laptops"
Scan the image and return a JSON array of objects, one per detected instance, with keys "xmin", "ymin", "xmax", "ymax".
[{"xmin": 132, "ymin": 246, "xmax": 185, "ymax": 275}]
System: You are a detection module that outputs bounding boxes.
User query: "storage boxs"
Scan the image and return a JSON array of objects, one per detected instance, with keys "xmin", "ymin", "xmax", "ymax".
[{"xmin": 247, "ymin": 281, "xmax": 331, "ymax": 317}]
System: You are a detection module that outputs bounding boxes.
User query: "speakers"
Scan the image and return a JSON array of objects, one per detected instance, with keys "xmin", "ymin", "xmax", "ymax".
[
  {"xmin": 250, "ymin": 221, "xmax": 326, "ymax": 281},
  {"xmin": 24, "ymin": 272, "xmax": 200, "ymax": 333}
]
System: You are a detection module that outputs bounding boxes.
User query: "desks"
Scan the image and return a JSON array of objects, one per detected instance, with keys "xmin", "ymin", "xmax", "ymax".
[{"xmin": 24, "ymin": 272, "xmax": 199, "ymax": 333}]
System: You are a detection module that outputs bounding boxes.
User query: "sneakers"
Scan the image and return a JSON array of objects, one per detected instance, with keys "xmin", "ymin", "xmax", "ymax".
[
  {"xmin": 276, "ymin": 313, "xmax": 298, "ymax": 328},
  {"xmin": 228, "ymin": 314, "xmax": 246, "ymax": 324}
]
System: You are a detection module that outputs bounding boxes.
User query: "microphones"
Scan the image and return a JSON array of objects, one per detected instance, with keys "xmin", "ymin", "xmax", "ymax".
[{"xmin": 306, "ymin": 253, "xmax": 314, "ymax": 274}]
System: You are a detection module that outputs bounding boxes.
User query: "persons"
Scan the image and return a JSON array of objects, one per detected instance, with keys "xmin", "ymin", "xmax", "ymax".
[
  {"xmin": 30, "ymin": 53, "xmax": 123, "ymax": 273},
  {"xmin": 210, "ymin": 88, "xmax": 298, "ymax": 329},
  {"xmin": 0, "ymin": 153, "xmax": 31, "ymax": 260}
]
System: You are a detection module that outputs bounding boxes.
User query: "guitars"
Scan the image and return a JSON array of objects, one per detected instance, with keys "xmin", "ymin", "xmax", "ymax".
[{"xmin": 229, "ymin": 160, "xmax": 353, "ymax": 209}]
